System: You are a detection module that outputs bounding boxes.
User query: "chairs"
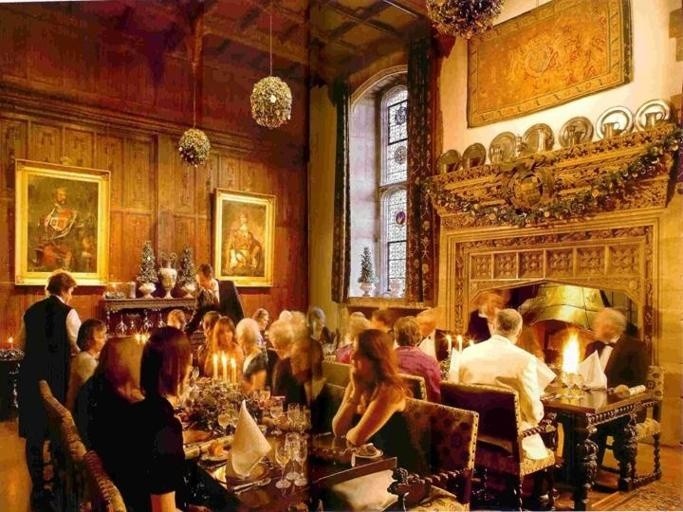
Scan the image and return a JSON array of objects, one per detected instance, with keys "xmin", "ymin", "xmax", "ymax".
[{"xmin": 0, "ymin": 323, "xmax": 668, "ymax": 512}]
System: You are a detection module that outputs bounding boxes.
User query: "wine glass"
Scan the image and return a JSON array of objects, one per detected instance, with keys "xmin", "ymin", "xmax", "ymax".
[
  {"xmin": 265, "ymin": 395, "xmax": 310, "ymax": 490},
  {"xmin": 561, "ymin": 368, "xmax": 589, "ymax": 400},
  {"xmin": 215, "ymin": 403, "xmax": 242, "ymax": 439}
]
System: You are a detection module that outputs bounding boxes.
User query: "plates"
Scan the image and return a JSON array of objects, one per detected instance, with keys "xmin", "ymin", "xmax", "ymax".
[
  {"xmin": 437, "ymin": 98, "xmax": 673, "ymax": 173},
  {"xmin": 180, "ymin": 428, "xmax": 267, "ymax": 482},
  {"xmin": 352, "ymin": 443, "xmax": 384, "ymax": 459}
]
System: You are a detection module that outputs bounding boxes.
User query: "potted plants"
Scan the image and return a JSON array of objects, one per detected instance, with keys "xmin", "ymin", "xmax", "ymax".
[
  {"xmin": 174, "ymin": 245, "xmax": 199, "ymax": 299},
  {"xmin": 356, "ymin": 243, "xmax": 380, "ymax": 296},
  {"xmin": 133, "ymin": 239, "xmax": 158, "ymax": 299}
]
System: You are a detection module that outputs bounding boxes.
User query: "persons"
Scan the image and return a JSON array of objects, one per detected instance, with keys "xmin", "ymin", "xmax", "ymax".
[
  {"xmin": 222, "ymin": 211, "xmax": 264, "ymax": 276},
  {"xmin": 66, "ymin": 308, "xmax": 453, "ymax": 512},
  {"xmin": 458, "ymin": 308, "xmax": 579, "ymax": 485},
  {"xmin": 462, "ymin": 293, "xmax": 504, "ymax": 351},
  {"xmin": 15, "ymin": 272, "xmax": 82, "ymax": 512},
  {"xmin": 35, "ymin": 185, "xmax": 90, "ymax": 273},
  {"xmin": 184, "ymin": 264, "xmax": 244, "ymax": 337},
  {"xmin": 583, "ymin": 307, "xmax": 650, "ymax": 485}
]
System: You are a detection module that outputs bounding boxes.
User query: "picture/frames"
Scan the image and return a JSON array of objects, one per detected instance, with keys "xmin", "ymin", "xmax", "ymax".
[
  {"xmin": 211, "ymin": 188, "xmax": 277, "ymax": 292},
  {"xmin": 10, "ymin": 155, "xmax": 115, "ymax": 289}
]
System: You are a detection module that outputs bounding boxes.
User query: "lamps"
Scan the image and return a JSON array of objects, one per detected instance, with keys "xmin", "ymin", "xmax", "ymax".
[
  {"xmin": 173, "ymin": 53, "xmax": 213, "ymax": 168},
  {"xmin": 421, "ymin": 1, "xmax": 507, "ymax": 40},
  {"xmin": 243, "ymin": 0, "xmax": 294, "ymax": 131}
]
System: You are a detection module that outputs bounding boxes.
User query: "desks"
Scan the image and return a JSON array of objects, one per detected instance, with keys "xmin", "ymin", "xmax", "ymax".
[{"xmin": 99, "ymin": 294, "xmax": 196, "ymax": 336}]
